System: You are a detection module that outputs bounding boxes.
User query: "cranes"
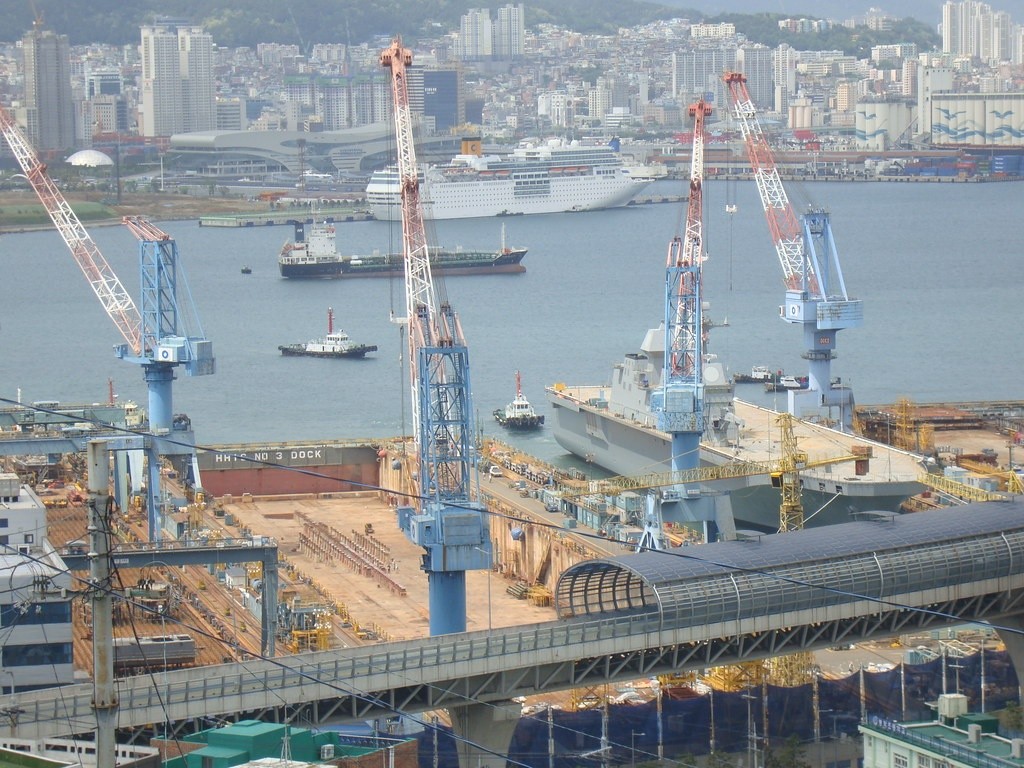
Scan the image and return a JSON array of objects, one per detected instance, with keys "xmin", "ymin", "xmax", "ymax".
[
  {"xmin": 555, "ymin": 414, "xmax": 873, "ymax": 533},
  {"xmin": 916, "ymin": 468, "xmax": 1024, "ymax": 500},
  {"xmin": 651, "ymin": 97, "xmax": 736, "ymax": 546},
  {"xmin": 380, "ymin": 35, "xmax": 493, "ymax": 636},
  {"xmin": 0, "ymin": 102, "xmax": 215, "ymax": 434},
  {"xmin": 722, "ymin": 69, "xmax": 854, "ymax": 423}
]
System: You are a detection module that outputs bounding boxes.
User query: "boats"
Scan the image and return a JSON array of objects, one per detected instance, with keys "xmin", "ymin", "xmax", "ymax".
[
  {"xmin": 0, "ymin": 378, "xmax": 191, "ymax": 443},
  {"xmin": 734, "ymin": 366, "xmax": 783, "ymax": 383},
  {"xmin": 279, "ymin": 307, "xmax": 378, "ymax": 360},
  {"xmin": 366, "ymin": 137, "xmax": 654, "ymax": 220},
  {"xmin": 766, "ymin": 376, "xmax": 841, "ymax": 391},
  {"xmin": 278, "ymin": 219, "xmax": 527, "ymax": 279},
  {"xmin": 493, "ymin": 371, "xmax": 544, "ymax": 429}
]
